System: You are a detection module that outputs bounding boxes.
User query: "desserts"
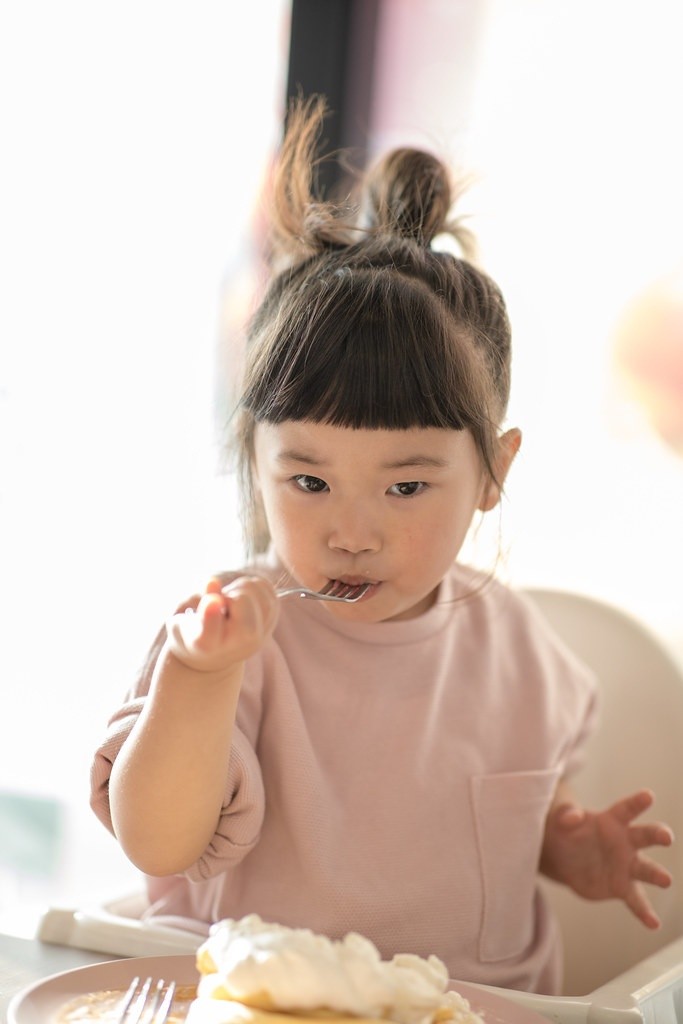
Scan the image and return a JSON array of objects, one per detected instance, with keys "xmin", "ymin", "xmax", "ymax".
[{"xmin": 192, "ymin": 913, "xmax": 483, "ymax": 1023}]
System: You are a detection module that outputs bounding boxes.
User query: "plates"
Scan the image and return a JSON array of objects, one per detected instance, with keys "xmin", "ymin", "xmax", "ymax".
[{"xmin": 6, "ymin": 955, "xmax": 554, "ymax": 1024}]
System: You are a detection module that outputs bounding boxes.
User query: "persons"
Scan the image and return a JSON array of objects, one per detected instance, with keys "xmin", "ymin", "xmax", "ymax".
[{"xmin": 88, "ymin": 101, "xmax": 676, "ymax": 997}]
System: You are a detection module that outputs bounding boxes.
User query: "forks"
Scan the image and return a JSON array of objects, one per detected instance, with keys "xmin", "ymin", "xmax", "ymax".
[
  {"xmin": 279, "ymin": 579, "xmax": 371, "ymax": 601},
  {"xmin": 114, "ymin": 976, "xmax": 176, "ymax": 1024}
]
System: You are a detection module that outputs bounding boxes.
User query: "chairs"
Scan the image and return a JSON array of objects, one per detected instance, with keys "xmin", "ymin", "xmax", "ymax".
[{"xmin": 37, "ymin": 586, "xmax": 683, "ymax": 1024}]
